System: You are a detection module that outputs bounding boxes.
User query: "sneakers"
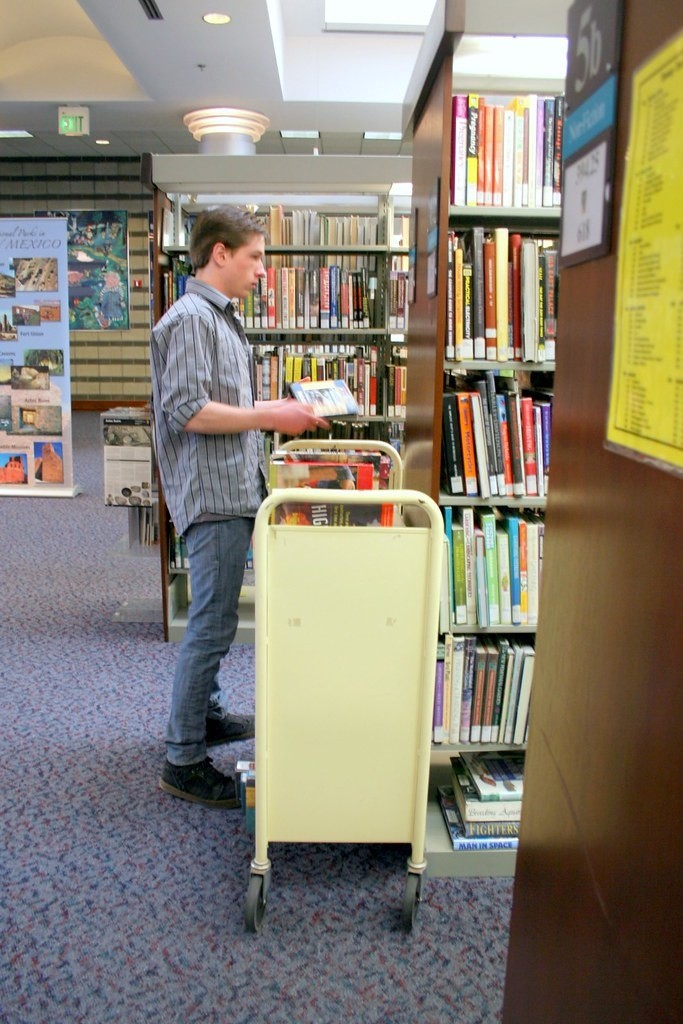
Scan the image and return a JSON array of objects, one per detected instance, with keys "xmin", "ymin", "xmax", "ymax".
[
  {"xmin": 205, "ymin": 704, "xmax": 256, "ymax": 747},
  {"xmin": 159, "ymin": 756, "xmax": 242, "ymax": 810}
]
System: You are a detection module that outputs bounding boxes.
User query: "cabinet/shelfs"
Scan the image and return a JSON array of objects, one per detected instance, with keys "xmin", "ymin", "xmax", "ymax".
[
  {"xmin": 137, "ymin": 150, "xmax": 413, "ymax": 646},
  {"xmin": 401, "ymin": 0, "xmax": 578, "ymax": 879},
  {"xmin": 244, "ymin": 438, "xmax": 444, "ymax": 936}
]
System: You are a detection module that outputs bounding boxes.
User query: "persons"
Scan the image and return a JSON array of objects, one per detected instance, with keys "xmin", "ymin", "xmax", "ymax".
[{"xmin": 149, "ymin": 204, "xmax": 330, "ymax": 807}]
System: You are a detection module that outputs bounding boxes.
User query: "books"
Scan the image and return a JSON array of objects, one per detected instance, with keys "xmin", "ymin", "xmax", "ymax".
[
  {"xmin": 289, "ymin": 380, "xmax": 359, "ymax": 417},
  {"xmin": 432, "ymin": 750, "xmax": 525, "ymax": 863},
  {"xmin": 164, "ymin": 93, "xmax": 563, "ymax": 743}
]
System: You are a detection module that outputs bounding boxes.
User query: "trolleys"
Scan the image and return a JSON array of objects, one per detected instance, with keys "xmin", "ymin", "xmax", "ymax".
[{"xmin": 243, "ymin": 438, "xmax": 443, "ymax": 936}]
